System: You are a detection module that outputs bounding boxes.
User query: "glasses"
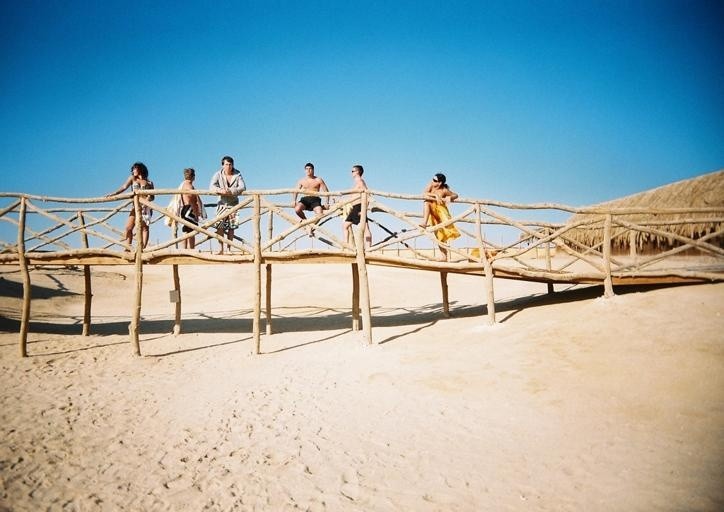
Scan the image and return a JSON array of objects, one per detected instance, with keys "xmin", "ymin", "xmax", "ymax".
[{"xmin": 433, "ymin": 179, "xmax": 439, "ymax": 182}]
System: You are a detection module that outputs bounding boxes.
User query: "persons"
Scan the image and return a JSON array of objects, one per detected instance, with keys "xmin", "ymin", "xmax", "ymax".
[
  {"xmin": 419, "ymin": 174, "xmax": 462, "ymax": 262},
  {"xmin": 343, "ymin": 166, "xmax": 372, "ymax": 248},
  {"xmin": 103, "ymin": 163, "xmax": 155, "ymax": 253},
  {"xmin": 292, "ymin": 163, "xmax": 330, "ymax": 237},
  {"xmin": 209, "ymin": 157, "xmax": 247, "ymax": 255},
  {"xmin": 180, "ymin": 169, "xmax": 202, "ymax": 249}
]
